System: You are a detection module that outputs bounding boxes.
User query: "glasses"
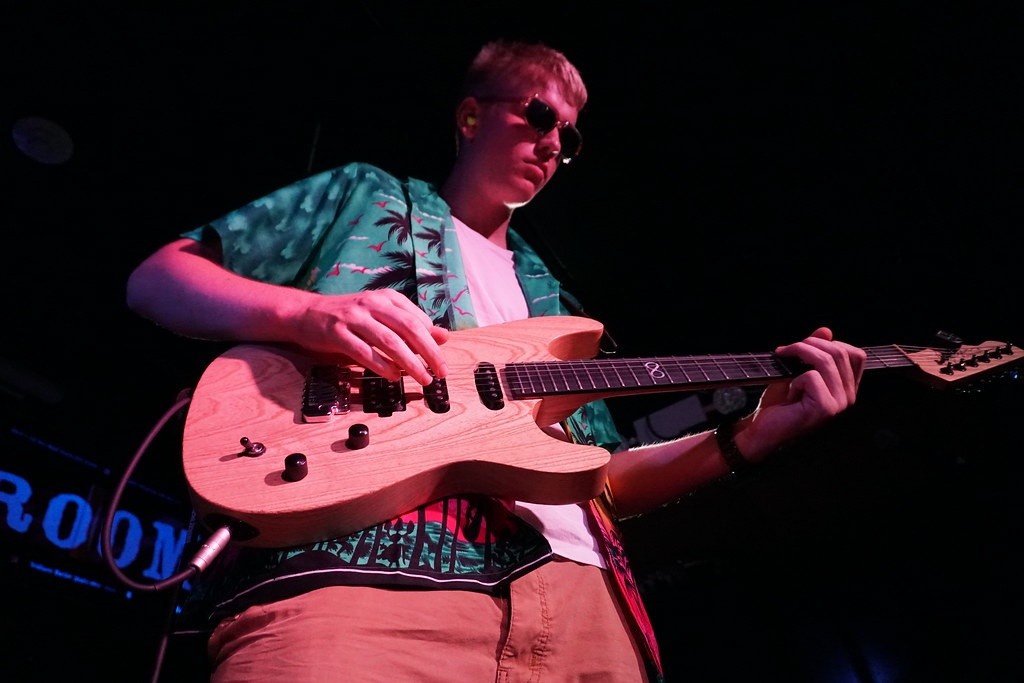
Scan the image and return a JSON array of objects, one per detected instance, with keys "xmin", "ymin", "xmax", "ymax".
[{"xmin": 477, "ymin": 93, "xmax": 584, "ymax": 164}]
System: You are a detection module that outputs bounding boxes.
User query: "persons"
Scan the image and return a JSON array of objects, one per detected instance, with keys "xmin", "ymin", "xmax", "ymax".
[{"xmin": 124, "ymin": 38, "xmax": 868, "ymax": 683}]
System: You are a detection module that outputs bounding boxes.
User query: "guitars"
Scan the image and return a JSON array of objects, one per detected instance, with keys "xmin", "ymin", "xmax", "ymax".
[{"xmin": 182, "ymin": 314, "xmax": 1024, "ymax": 549}]
